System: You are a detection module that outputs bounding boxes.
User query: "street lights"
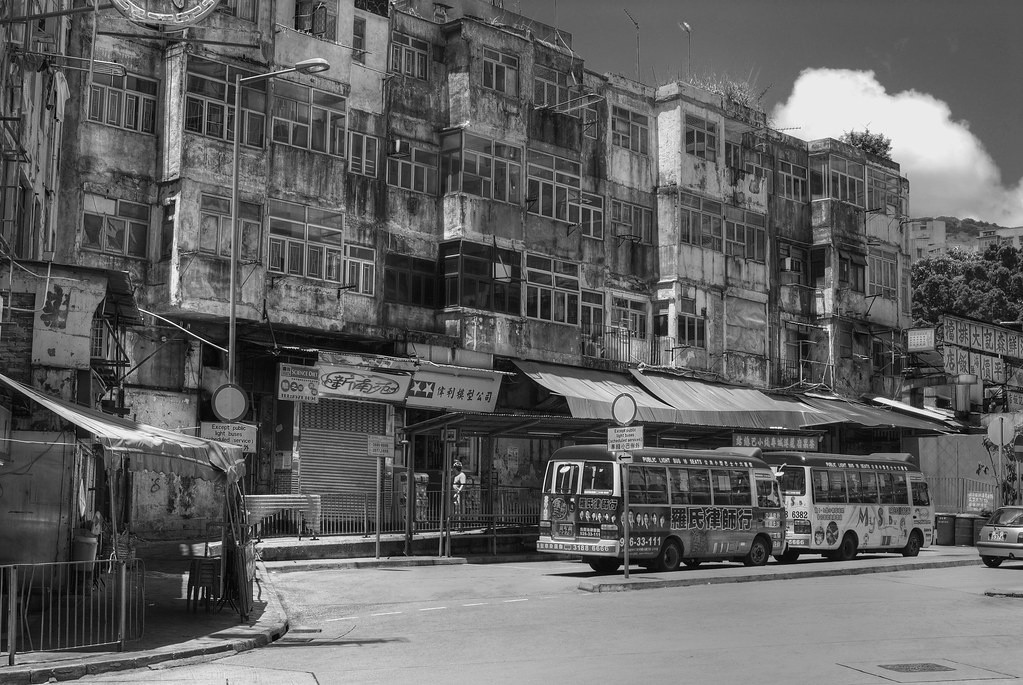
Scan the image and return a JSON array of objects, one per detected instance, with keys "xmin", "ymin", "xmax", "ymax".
[{"xmin": 227, "ymin": 56, "xmax": 332, "ymax": 385}]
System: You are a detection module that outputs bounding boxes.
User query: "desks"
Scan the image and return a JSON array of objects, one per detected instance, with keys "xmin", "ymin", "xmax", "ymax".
[{"xmin": 243, "ymin": 494, "xmax": 321, "ymax": 543}]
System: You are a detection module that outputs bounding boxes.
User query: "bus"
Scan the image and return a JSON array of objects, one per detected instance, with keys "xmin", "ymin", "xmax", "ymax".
[
  {"xmin": 535, "ymin": 444, "xmax": 787, "ymax": 574},
  {"xmin": 761, "ymin": 451, "xmax": 939, "ymax": 565}
]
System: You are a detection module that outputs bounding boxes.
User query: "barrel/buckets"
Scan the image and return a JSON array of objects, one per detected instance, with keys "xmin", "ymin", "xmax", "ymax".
[
  {"xmin": 974, "ymin": 518, "xmax": 991, "ymax": 546},
  {"xmin": 73, "ymin": 536, "xmax": 99, "ymax": 571},
  {"xmin": 937, "ymin": 514, "xmax": 956, "ymax": 546},
  {"xmin": 955, "ymin": 517, "xmax": 973, "ymax": 546}
]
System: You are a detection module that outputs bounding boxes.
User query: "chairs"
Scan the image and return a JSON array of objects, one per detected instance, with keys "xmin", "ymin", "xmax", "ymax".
[
  {"xmin": 815, "ymin": 490, "xmax": 893, "ymax": 503},
  {"xmin": 647, "ymin": 484, "xmax": 667, "ymax": 503},
  {"xmin": 186, "ymin": 558, "xmax": 223, "ymax": 613},
  {"xmin": 673, "ymin": 487, "xmax": 747, "ymax": 504},
  {"xmin": 628, "ymin": 485, "xmax": 645, "ymax": 505}
]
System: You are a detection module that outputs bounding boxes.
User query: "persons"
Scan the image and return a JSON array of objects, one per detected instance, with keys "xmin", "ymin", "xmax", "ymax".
[
  {"xmin": 617, "ymin": 509, "xmax": 667, "ymax": 528},
  {"xmin": 451, "ymin": 461, "xmax": 467, "ymax": 530},
  {"xmin": 578, "ymin": 509, "xmax": 616, "ymax": 523}
]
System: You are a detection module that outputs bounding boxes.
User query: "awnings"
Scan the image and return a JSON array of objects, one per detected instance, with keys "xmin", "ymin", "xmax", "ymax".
[
  {"xmin": 511, "ymin": 358, "xmax": 676, "ymax": 422},
  {"xmin": 795, "ymin": 395, "xmax": 960, "ymax": 432},
  {"xmin": 0, "ymin": 374, "xmax": 244, "ymax": 480},
  {"xmin": 629, "ymin": 367, "xmax": 846, "ymax": 431}
]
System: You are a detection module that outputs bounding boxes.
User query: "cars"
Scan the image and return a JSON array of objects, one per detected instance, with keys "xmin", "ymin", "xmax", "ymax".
[{"xmin": 975, "ymin": 505, "xmax": 1023, "ymax": 568}]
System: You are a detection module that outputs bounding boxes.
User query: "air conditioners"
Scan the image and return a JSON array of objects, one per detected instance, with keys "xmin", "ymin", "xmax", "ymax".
[
  {"xmin": 778, "ymin": 257, "xmax": 803, "ymax": 274},
  {"xmin": 582, "ymin": 341, "xmax": 601, "ymax": 359},
  {"xmin": 388, "ymin": 140, "xmax": 412, "ymax": 158}
]
